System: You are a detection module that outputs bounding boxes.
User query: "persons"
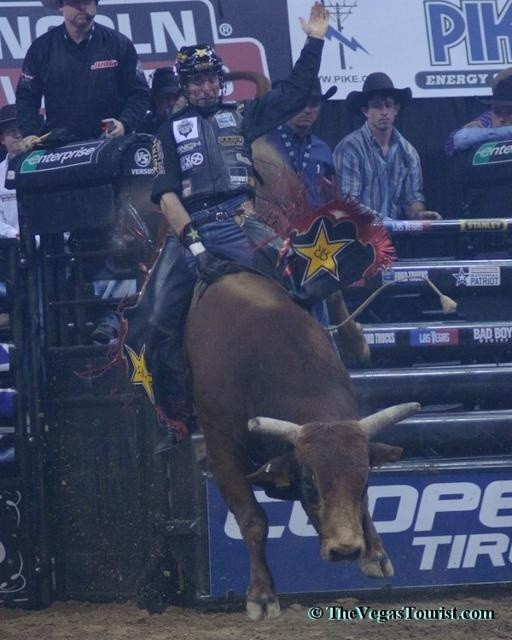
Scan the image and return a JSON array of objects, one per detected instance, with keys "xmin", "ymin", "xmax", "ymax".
[
  {"xmin": 331, "ymin": 72, "xmax": 444, "ymax": 259},
  {"xmin": 445, "ymin": 66, "xmax": 512, "ymax": 258}
]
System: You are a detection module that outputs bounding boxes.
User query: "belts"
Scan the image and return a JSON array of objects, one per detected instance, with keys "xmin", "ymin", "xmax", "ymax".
[{"xmin": 183, "ymin": 190, "xmax": 246, "ymax": 214}]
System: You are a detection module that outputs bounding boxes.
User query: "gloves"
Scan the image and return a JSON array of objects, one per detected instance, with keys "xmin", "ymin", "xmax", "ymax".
[{"xmin": 188, "ymin": 241, "xmax": 230, "ymax": 285}]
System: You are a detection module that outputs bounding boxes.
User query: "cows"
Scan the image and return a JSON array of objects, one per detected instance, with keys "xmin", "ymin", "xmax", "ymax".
[{"xmin": 158, "ymin": 70, "xmax": 422, "ymax": 623}]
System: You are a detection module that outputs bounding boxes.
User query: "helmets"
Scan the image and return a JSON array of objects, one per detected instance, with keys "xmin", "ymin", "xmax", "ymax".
[{"xmin": 173, "ymin": 39, "xmax": 228, "ymax": 120}]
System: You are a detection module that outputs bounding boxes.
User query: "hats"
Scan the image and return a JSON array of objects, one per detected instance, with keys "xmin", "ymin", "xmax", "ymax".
[
  {"xmin": 345, "ymin": 70, "xmax": 428, "ymax": 118},
  {"xmin": 147, "ymin": 66, "xmax": 185, "ymax": 97},
  {"xmin": 479, "ymin": 75, "xmax": 512, "ymax": 108},
  {"xmin": 271, "ymin": 73, "xmax": 339, "ymax": 101}
]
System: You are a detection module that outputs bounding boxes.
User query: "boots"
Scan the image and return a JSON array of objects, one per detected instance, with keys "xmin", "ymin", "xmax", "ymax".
[{"xmin": 150, "ymin": 331, "xmax": 200, "ymax": 456}]
